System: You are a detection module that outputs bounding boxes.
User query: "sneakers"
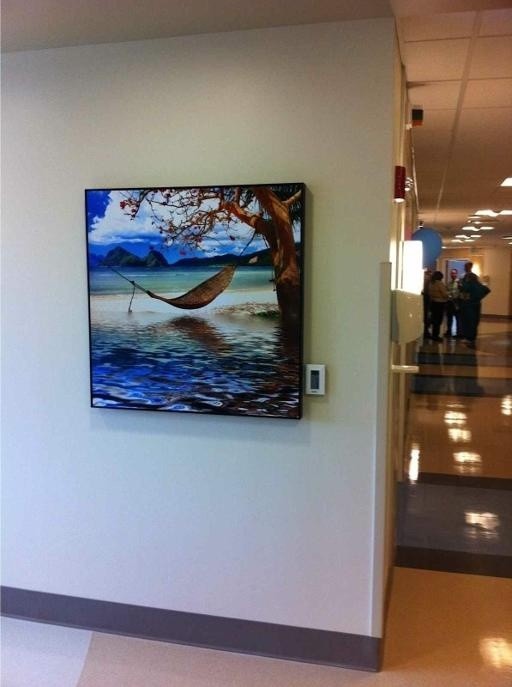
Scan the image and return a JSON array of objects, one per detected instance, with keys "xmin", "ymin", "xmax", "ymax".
[{"xmin": 423, "ymin": 330, "xmax": 464, "ymax": 343}]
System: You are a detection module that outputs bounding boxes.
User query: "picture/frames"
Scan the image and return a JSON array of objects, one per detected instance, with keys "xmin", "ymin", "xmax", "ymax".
[{"xmin": 84, "ymin": 182, "xmax": 305, "ymax": 420}]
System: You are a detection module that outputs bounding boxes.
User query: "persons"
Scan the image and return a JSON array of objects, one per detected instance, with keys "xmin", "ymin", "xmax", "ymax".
[{"xmin": 425, "ymin": 259, "xmax": 481, "ymax": 344}]
